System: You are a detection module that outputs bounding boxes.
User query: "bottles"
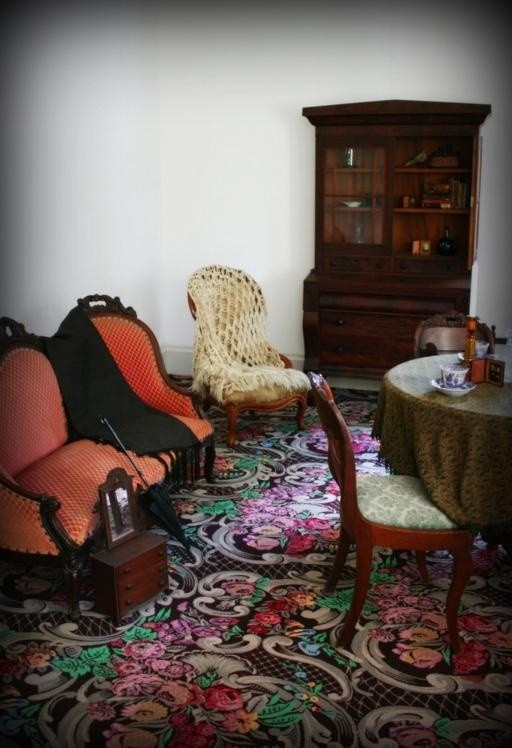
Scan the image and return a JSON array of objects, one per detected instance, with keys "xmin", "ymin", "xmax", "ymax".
[
  {"xmin": 344, "ymin": 147, "xmax": 355, "ymax": 167},
  {"xmin": 437, "ymin": 225, "xmax": 457, "ymax": 254}
]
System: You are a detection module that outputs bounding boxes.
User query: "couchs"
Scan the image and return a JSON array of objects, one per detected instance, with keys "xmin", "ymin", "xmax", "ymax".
[{"xmin": 1, "ymin": 293, "xmax": 215, "ymax": 623}]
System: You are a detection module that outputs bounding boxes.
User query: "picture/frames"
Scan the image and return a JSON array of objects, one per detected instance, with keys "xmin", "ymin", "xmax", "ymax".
[{"xmin": 99, "ymin": 468, "xmax": 141, "ymax": 551}]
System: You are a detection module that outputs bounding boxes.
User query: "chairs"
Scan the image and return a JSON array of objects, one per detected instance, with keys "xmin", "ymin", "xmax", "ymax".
[
  {"xmin": 184, "ymin": 266, "xmax": 313, "ymax": 448},
  {"xmin": 413, "ymin": 309, "xmax": 497, "ymax": 359},
  {"xmin": 308, "ymin": 368, "xmax": 473, "ymax": 652}
]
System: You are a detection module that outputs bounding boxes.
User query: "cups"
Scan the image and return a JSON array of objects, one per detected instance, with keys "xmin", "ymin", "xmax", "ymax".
[
  {"xmin": 475, "ymin": 340, "xmax": 490, "ymax": 357},
  {"xmin": 440, "ymin": 361, "xmax": 470, "ymax": 385}
]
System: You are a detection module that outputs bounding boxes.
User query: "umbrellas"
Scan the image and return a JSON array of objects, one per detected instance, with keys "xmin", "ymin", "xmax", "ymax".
[{"xmin": 98, "ymin": 416, "xmax": 192, "ymax": 552}]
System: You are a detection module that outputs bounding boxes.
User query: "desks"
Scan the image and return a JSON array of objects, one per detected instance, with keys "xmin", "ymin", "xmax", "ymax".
[{"xmin": 370, "ymin": 353, "xmax": 512, "ymax": 550}]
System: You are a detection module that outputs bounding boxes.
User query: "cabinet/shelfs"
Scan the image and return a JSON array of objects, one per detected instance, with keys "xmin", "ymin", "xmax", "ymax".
[
  {"xmin": 90, "ymin": 534, "xmax": 170, "ymax": 625},
  {"xmin": 301, "ymin": 98, "xmax": 493, "ymax": 408}
]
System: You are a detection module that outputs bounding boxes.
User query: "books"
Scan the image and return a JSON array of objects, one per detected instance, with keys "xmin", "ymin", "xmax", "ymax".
[{"xmin": 449, "ymin": 177, "xmax": 468, "ymax": 210}]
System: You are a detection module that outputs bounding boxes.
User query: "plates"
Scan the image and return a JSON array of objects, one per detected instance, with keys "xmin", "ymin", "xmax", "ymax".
[
  {"xmin": 431, "ymin": 377, "xmax": 477, "ymax": 397},
  {"xmin": 457, "ymin": 350, "xmax": 498, "ymax": 361}
]
